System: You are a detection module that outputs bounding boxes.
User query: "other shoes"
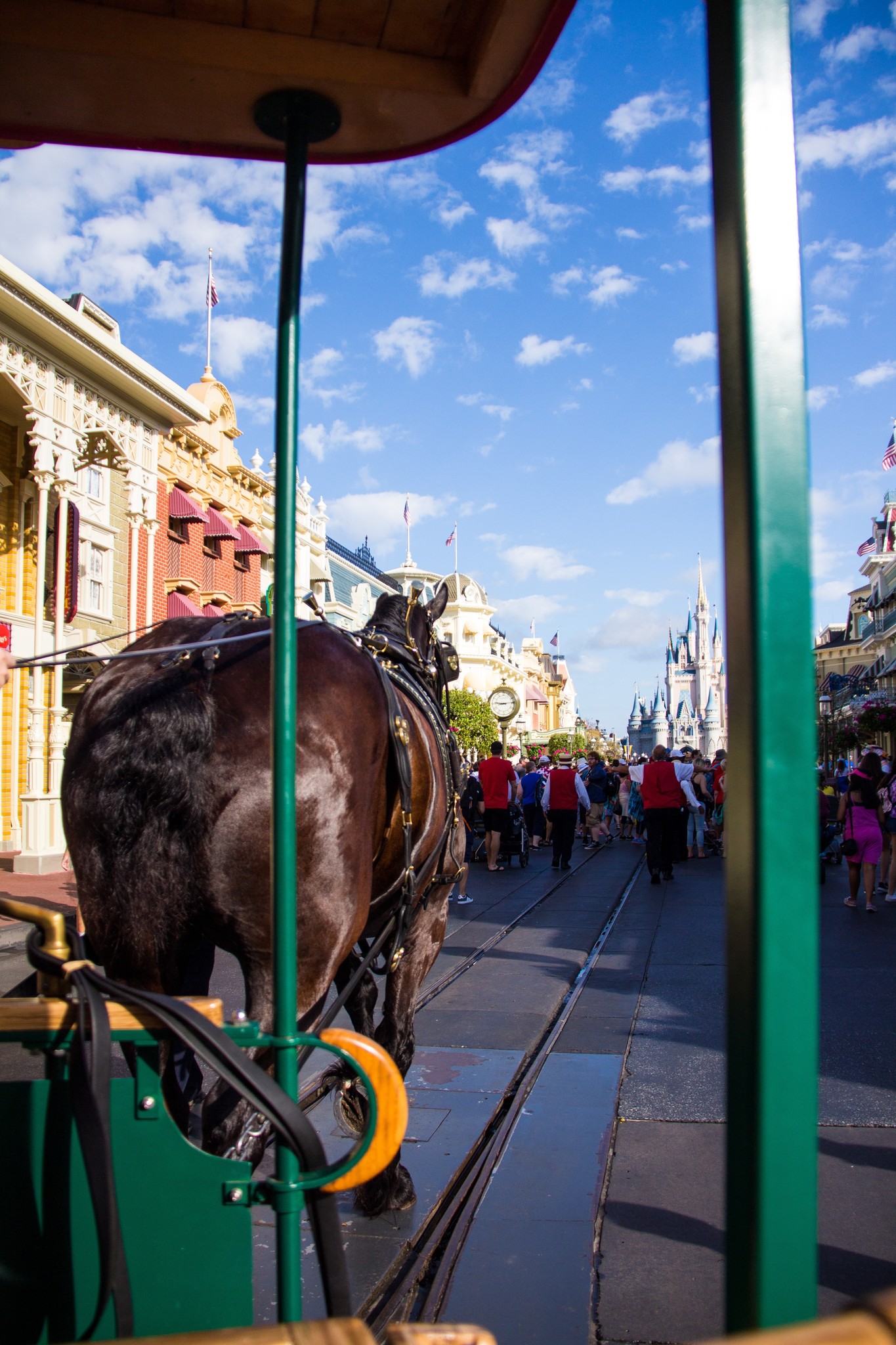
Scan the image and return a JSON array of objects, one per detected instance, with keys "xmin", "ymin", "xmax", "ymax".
[
  {"xmin": 651, "ymin": 875, "xmax": 661, "ymax": 884},
  {"xmin": 688, "ymin": 841, "xmax": 726, "ymax": 858},
  {"xmin": 662, "ymin": 875, "xmax": 674, "ymax": 880}
]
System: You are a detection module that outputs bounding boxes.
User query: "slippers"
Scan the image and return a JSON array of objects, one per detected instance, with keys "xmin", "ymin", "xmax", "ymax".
[{"xmin": 490, "ymin": 865, "xmax": 504, "ymax": 871}]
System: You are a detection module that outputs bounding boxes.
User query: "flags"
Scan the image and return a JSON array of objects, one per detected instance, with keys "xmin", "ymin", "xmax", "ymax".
[
  {"xmin": 404, "ymin": 500, "xmax": 412, "ymax": 527},
  {"xmin": 530, "ymin": 620, "xmax": 534, "ymax": 634},
  {"xmin": 882, "ymin": 426, "xmax": 896, "ymax": 471},
  {"xmin": 550, "ymin": 630, "xmax": 559, "ymax": 646},
  {"xmin": 446, "ymin": 526, "xmax": 456, "ymax": 546},
  {"xmin": 206, "ymin": 260, "xmax": 220, "ymax": 309},
  {"xmin": 856, "ymin": 537, "xmax": 877, "ymax": 557}
]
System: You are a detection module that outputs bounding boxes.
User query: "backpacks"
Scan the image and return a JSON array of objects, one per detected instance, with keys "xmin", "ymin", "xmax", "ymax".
[
  {"xmin": 585, "ymin": 762, "xmax": 621, "ymax": 797},
  {"xmin": 876, "ymin": 775, "xmax": 896, "ymax": 818},
  {"xmin": 703, "ymin": 764, "xmax": 721, "ymax": 801},
  {"xmin": 535, "ymin": 774, "xmax": 547, "ymax": 801}
]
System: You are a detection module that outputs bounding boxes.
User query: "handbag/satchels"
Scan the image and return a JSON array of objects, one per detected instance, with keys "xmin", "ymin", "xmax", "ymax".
[
  {"xmin": 713, "ymin": 802, "xmax": 723, "ymax": 825},
  {"xmin": 612, "ymin": 798, "xmax": 622, "ymax": 816},
  {"xmin": 840, "ymin": 838, "xmax": 856, "ymax": 856},
  {"xmin": 845, "ymin": 778, "xmax": 850, "ymax": 784}
]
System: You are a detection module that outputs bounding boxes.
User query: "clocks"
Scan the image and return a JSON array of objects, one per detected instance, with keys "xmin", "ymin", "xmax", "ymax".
[{"xmin": 488, "ymin": 688, "xmax": 520, "ymax": 720}]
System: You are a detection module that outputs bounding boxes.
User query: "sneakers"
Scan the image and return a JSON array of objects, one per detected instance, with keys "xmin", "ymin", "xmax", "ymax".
[
  {"xmin": 457, "ymin": 894, "xmax": 473, "ymax": 904},
  {"xmin": 448, "ymin": 891, "xmax": 453, "ymax": 901},
  {"xmin": 843, "ymin": 881, "xmax": 896, "ymax": 912},
  {"xmin": 529, "ymin": 824, "xmax": 647, "ymax": 869}
]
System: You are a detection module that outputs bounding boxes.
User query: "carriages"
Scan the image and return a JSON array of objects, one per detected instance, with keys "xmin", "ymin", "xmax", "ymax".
[{"xmin": 0, "ymin": 1, "xmax": 837, "ymax": 1345}]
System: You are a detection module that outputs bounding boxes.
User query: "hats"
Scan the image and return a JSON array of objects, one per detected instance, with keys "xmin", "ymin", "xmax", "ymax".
[
  {"xmin": 705, "ymin": 759, "xmax": 710, "ymax": 763},
  {"xmin": 619, "ymin": 759, "xmax": 627, "ymax": 764},
  {"xmin": 715, "ymin": 749, "xmax": 727, "ymax": 760},
  {"xmin": 882, "ymin": 751, "xmax": 890, "ymax": 759},
  {"xmin": 670, "ymin": 749, "xmax": 685, "ymax": 757},
  {"xmin": 526, "ymin": 754, "xmax": 590, "ymax": 773},
  {"xmin": 666, "ymin": 748, "xmax": 671, "ymax": 753}
]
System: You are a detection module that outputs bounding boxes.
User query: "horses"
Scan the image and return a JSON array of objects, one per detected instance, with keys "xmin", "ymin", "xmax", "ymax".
[{"xmin": 60, "ymin": 585, "xmax": 468, "ymax": 1217}]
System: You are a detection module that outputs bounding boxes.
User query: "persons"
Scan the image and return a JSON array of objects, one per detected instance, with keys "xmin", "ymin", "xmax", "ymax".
[
  {"xmin": 834, "ymin": 767, "xmax": 886, "ymax": 914},
  {"xmin": 602, "ymin": 744, "xmax": 718, "ymax": 885},
  {"xmin": 817, "ymin": 788, "xmax": 832, "ymax": 885},
  {"xmin": 684, "ymin": 758, "xmax": 709, "ymax": 859},
  {"xmin": 582, "ymin": 751, "xmax": 616, "ymax": 851},
  {"xmin": 447, "ymin": 758, "xmax": 486, "ymax": 905},
  {"xmin": 461, "ymin": 748, "xmax": 728, "ymax": 844},
  {"xmin": 719, "ymin": 757, "xmax": 729, "ymax": 858},
  {"xmin": 875, "ymin": 755, "xmax": 896, "ymax": 903},
  {"xmin": 814, "ymin": 742, "xmax": 894, "ymax": 825},
  {"xmin": 478, "ymin": 741, "xmax": 518, "ymax": 872},
  {"xmin": 516, "ymin": 761, "xmax": 544, "ymax": 851},
  {"xmin": 540, "ymin": 753, "xmax": 592, "ymax": 870}
]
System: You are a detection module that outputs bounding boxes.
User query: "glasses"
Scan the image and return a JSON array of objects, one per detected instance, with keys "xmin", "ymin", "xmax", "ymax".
[
  {"xmin": 697, "ymin": 754, "xmax": 702, "ymax": 756},
  {"xmin": 521, "ymin": 760, "xmax": 527, "ymax": 764},
  {"xmin": 587, "ymin": 757, "xmax": 596, "ymax": 761}
]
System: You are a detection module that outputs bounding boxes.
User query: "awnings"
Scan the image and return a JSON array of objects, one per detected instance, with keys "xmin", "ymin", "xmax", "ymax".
[
  {"xmin": 169, "ymin": 487, "xmax": 269, "ymax": 556},
  {"xmin": 525, "ymin": 684, "xmax": 550, "ymax": 705},
  {"xmin": 817, "ymin": 654, "xmax": 896, "ymax": 692},
  {"xmin": 167, "ymin": 591, "xmax": 226, "ymax": 619}
]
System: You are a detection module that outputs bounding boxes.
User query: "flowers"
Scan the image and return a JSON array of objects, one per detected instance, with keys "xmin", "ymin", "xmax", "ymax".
[
  {"xmin": 826, "ymin": 699, "xmax": 896, "ymax": 752},
  {"xmin": 507, "ymin": 743, "xmax": 618, "ymax": 763}
]
